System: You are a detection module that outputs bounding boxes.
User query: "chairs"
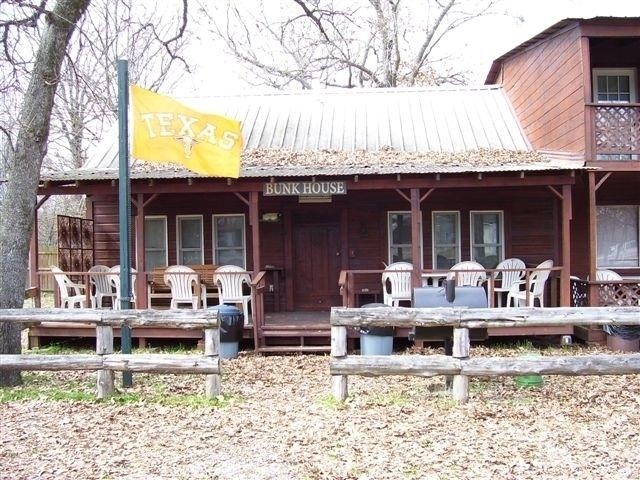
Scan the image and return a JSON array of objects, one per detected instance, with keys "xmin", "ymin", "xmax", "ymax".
[
  {"xmin": 379, "ymin": 257, "xmax": 555, "ymax": 308},
  {"xmin": 48, "ymin": 262, "xmax": 257, "ymax": 326},
  {"xmin": 569, "ymin": 269, "xmax": 632, "ymax": 307}
]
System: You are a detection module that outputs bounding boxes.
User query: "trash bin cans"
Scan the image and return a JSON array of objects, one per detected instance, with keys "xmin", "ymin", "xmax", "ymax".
[
  {"xmin": 218, "ymin": 305, "xmax": 241, "ymax": 359},
  {"xmin": 606, "ymin": 325, "xmax": 640, "ymax": 352},
  {"xmin": 360, "ymin": 303, "xmax": 394, "ymax": 355}
]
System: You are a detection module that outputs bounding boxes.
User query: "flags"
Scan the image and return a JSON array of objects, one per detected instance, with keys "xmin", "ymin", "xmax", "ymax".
[{"xmin": 131, "ymin": 83, "xmax": 243, "ymax": 180}]
